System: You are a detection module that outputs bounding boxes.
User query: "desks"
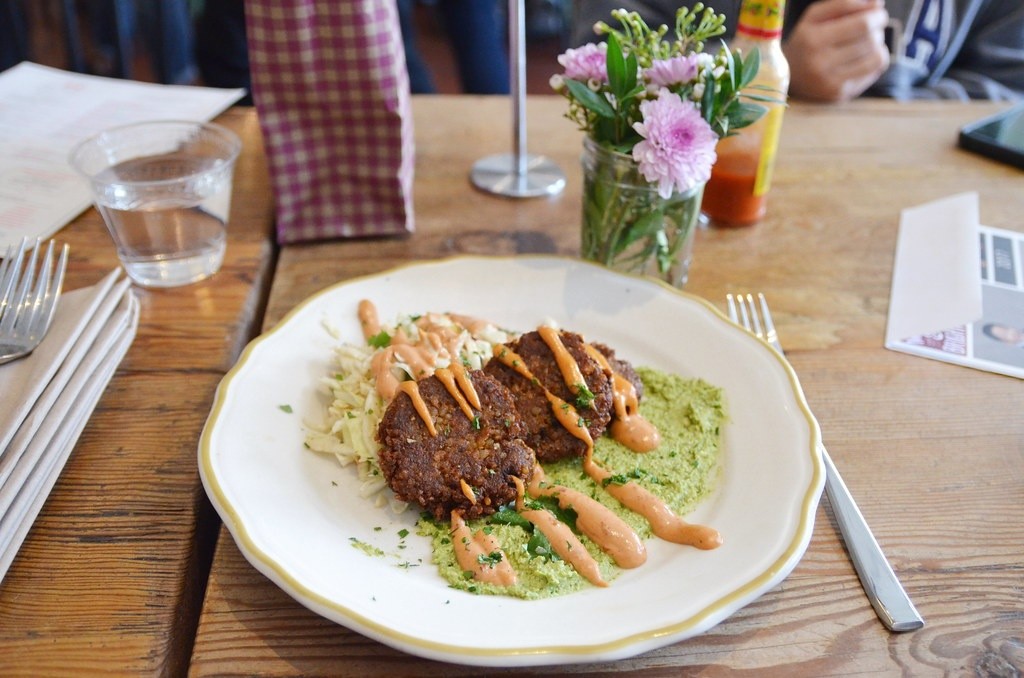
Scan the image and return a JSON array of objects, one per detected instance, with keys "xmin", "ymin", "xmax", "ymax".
[{"xmin": 0, "ymin": 100, "xmax": 1024, "ymax": 678}]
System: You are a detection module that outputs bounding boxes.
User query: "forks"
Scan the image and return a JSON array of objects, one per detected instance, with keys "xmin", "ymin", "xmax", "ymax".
[
  {"xmin": 724, "ymin": 292, "xmax": 926, "ymax": 633},
  {"xmin": 0, "ymin": 234, "xmax": 68, "ymax": 364}
]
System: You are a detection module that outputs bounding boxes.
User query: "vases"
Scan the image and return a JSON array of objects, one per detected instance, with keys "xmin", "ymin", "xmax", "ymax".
[{"xmin": 577, "ymin": 138, "xmax": 707, "ymax": 290}]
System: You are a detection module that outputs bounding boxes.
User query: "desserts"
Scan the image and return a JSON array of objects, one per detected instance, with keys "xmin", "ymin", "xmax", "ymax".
[
  {"xmin": 590, "ymin": 343, "xmax": 643, "ymax": 410},
  {"xmin": 382, "ymin": 372, "xmax": 534, "ymax": 512},
  {"xmin": 486, "ymin": 330, "xmax": 611, "ymax": 455}
]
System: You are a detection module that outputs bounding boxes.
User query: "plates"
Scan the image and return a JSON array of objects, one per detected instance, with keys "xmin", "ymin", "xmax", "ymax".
[
  {"xmin": 0, "ymin": 267, "xmax": 142, "ymax": 586},
  {"xmin": 196, "ymin": 252, "xmax": 828, "ymax": 666}
]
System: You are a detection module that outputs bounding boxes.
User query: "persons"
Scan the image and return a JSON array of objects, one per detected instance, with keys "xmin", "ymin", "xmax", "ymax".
[
  {"xmin": 568, "ymin": 1, "xmax": 1024, "ymax": 107},
  {"xmin": 399, "ymin": 0, "xmax": 510, "ymax": 97}
]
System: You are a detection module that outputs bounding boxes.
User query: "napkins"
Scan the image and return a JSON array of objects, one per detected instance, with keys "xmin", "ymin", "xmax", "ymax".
[{"xmin": 0, "ymin": 60, "xmax": 246, "ymax": 587}]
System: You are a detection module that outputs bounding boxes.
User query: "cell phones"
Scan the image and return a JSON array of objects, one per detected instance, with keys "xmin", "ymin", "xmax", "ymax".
[{"xmin": 959, "ymin": 105, "xmax": 1024, "ymax": 171}]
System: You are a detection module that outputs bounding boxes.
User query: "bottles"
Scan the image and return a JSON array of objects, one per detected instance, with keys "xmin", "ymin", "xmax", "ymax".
[{"xmin": 700, "ymin": 0, "xmax": 791, "ymax": 234}]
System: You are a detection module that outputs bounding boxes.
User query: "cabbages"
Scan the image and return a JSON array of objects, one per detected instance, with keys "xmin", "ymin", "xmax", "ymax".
[{"xmin": 306, "ymin": 314, "xmax": 486, "ymax": 469}]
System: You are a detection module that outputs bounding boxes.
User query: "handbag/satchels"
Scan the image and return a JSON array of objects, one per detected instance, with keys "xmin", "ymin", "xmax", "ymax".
[{"xmin": 246, "ymin": 0, "xmax": 417, "ymax": 245}]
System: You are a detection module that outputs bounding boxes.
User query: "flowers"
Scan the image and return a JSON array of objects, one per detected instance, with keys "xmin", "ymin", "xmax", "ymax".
[{"xmin": 549, "ymin": 2, "xmax": 789, "ymax": 198}]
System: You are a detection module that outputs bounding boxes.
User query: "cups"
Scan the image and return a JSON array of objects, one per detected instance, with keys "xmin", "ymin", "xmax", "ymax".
[{"xmin": 69, "ymin": 119, "xmax": 243, "ymax": 289}]
